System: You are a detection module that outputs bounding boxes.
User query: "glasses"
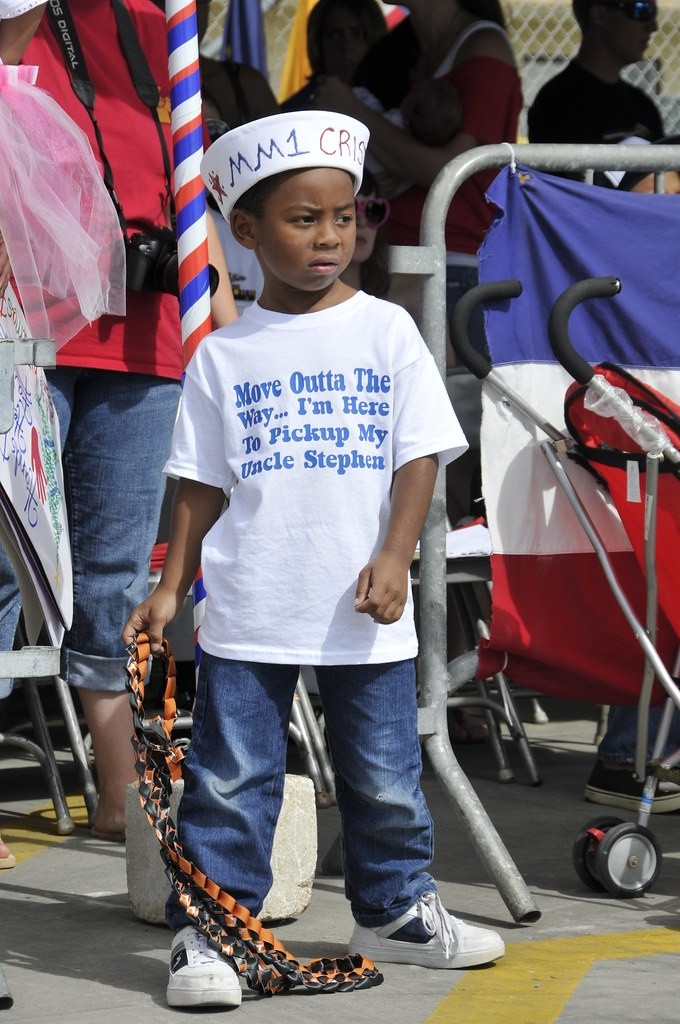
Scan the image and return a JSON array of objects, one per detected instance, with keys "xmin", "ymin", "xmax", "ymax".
[
  {"xmin": 608, "ymin": 0, "xmax": 657, "ymax": 21},
  {"xmin": 356, "ymin": 195, "xmax": 390, "ymax": 228}
]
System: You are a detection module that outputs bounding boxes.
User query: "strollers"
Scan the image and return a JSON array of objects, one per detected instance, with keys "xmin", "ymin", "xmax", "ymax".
[{"xmin": 450, "ymin": 280, "xmax": 680, "ymax": 893}]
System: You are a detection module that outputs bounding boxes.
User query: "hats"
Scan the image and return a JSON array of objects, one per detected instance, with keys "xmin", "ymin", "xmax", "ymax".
[{"xmin": 199, "ymin": 109, "xmax": 370, "ymax": 222}]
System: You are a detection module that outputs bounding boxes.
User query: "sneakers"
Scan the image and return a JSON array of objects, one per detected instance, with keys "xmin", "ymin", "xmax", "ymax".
[
  {"xmin": 347, "ymin": 899, "xmax": 509, "ymax": 968},
  {"xmin": 585, "ymin": 748, "xmax": 679, "ymax": 812},
  {"xmin": 165, "ymin": 919, "xmax": 248, "ymax": 1008}
]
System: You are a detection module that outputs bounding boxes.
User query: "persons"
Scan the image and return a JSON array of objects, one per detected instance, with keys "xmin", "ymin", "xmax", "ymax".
[
  {"xmin": 194, "ymin": 1, "xmax": 524, "ymax": 743},
  {"xmin": 121, "ymin": 110, "xmax": 504, "ymax": 1009},
  {"xmin": 0, "ymin": 1, "xmax": 237, "ymax": 870},
  {"xmin": 526, "ymin": 0, "xmax": 679, "ymax": 193},
  {"xmin": 585, "ymin": 705, "xmax": 680, "ymax": 812}
]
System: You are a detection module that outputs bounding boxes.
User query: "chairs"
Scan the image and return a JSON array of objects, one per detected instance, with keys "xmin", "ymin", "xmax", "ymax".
[{"xmin": 1, "ymin": 547, "xmax": 613, "ymax": 835}]
